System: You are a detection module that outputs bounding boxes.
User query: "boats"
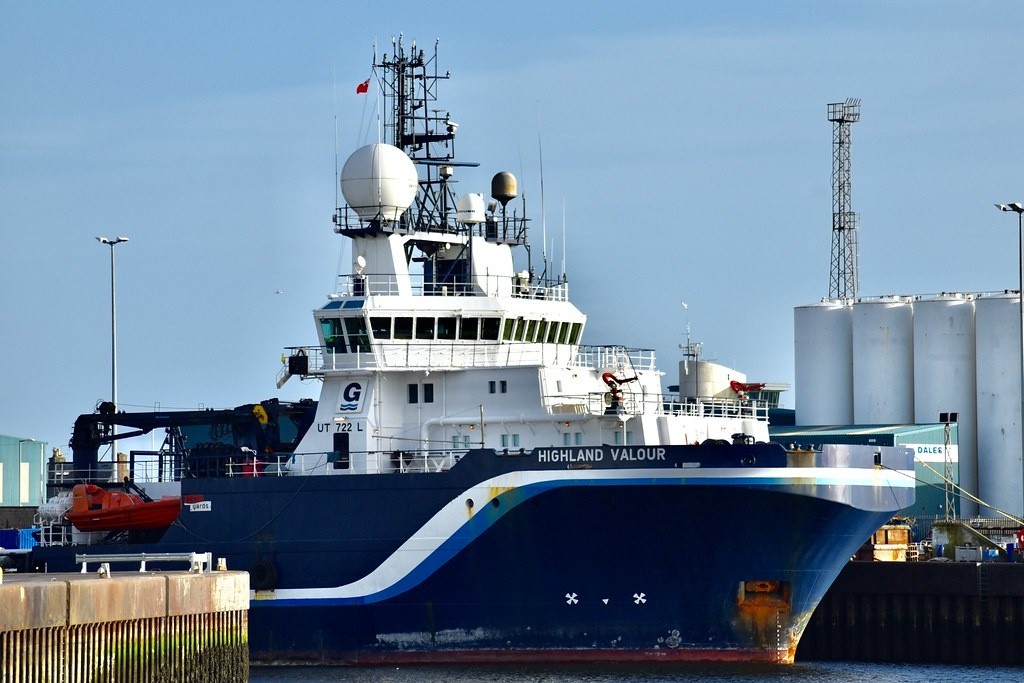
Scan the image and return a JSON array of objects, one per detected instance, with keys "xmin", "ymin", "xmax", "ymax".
[
  {"xmin": 66, "ymin": 483, "xmax": 182, "ymax": 532},
  {"xmin": 17, "ymin": 29, "xmax": 917, "ymax": 676}
]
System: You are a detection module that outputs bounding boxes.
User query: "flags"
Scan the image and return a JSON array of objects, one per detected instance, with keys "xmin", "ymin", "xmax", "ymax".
[{"xmin": 357, "ymin": 79, "xmax": 370, "ymax": 94}]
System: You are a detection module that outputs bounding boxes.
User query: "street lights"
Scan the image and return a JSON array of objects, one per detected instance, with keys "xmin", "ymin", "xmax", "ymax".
[
  {"xmin": 993, "ymin": 202, "xmax": 1024, "ymax": 498},
  {"xmin": 19, "ymin": 438, "xmax": 37, "ymax": 507},
  {"xmin": 96, "ymin": 235, "xmax": 131, "ymax": 484}
]
